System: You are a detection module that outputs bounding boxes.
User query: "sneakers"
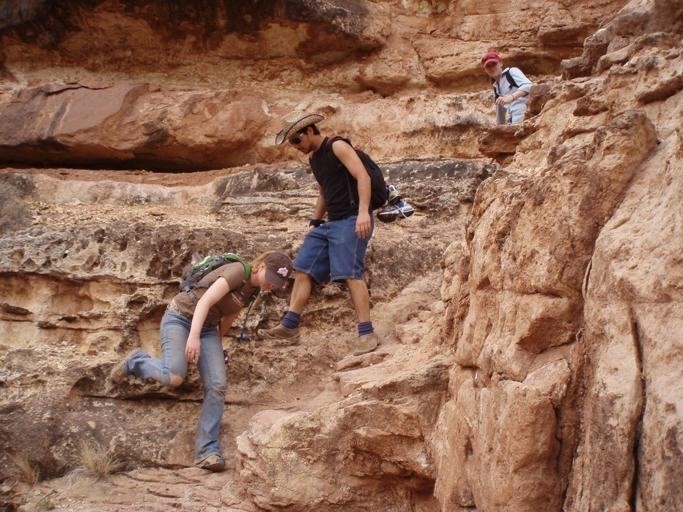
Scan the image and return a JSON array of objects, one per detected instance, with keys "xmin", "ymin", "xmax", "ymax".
[
  {"xmin": 257, "ymin": 323, "xmax": 302, "ymax": 346},
  {"xmin": 354, "ymin": 331, "xmax": 380, "ymax": 356},
  {"xmin": 377, "ymin": 199, "xmax": 415, "ymax": 221},
  {"xmin": 197, "ymin": 454, "xmax": 225, "ymax": 473},
  {"xmin": 110, "ymin": 349, "xmax": 144, "ymax": 384}
]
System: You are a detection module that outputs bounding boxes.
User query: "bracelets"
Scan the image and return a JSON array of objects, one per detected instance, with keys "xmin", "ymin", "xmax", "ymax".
[
  {"xmin": 309, "ymin": 220, "xmax": 320, "ymax": 228},
  {"xmin": 511, "ymin": 95, "xmax": 514, "ymax": 101}
]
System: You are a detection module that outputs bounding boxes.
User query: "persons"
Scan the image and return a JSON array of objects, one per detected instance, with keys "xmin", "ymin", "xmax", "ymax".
[
  {"xmin": 480, "ymin": 52, "xmax": 533, "ymax": 124},
  {"xmin": 257, "ymin": 110, "xmax": 380, "ymax": 357},
  {"xmin": 110, "ymin": 251, "xmax": 292, "ymax": 470}
]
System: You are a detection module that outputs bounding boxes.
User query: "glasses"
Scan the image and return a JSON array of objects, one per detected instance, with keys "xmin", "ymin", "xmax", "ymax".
[{"xmin": 287, "ymin": 130, "xmax": 304, "ymax": 144}]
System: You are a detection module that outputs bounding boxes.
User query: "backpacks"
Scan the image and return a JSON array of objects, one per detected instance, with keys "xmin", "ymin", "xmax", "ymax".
[
  {"xmin": 350, "ymin": 151, "xmax": 388, "ymax": 211},
  {"xmin": 177, "ymin": 251, "xmax": 240, "ymax": 293}
]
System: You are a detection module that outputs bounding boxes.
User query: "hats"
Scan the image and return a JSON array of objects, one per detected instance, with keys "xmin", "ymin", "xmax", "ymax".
[
  {"xmin": 263, "ymin": 250, "xmax": 293, "ymax": 288},
  {"xmin": 275, "ymin": 111, "xmax": 325, "ymax": 145},
  {"xmin": 480, "ymin": 53, "xmax": 501, "ymax": 69}
]
